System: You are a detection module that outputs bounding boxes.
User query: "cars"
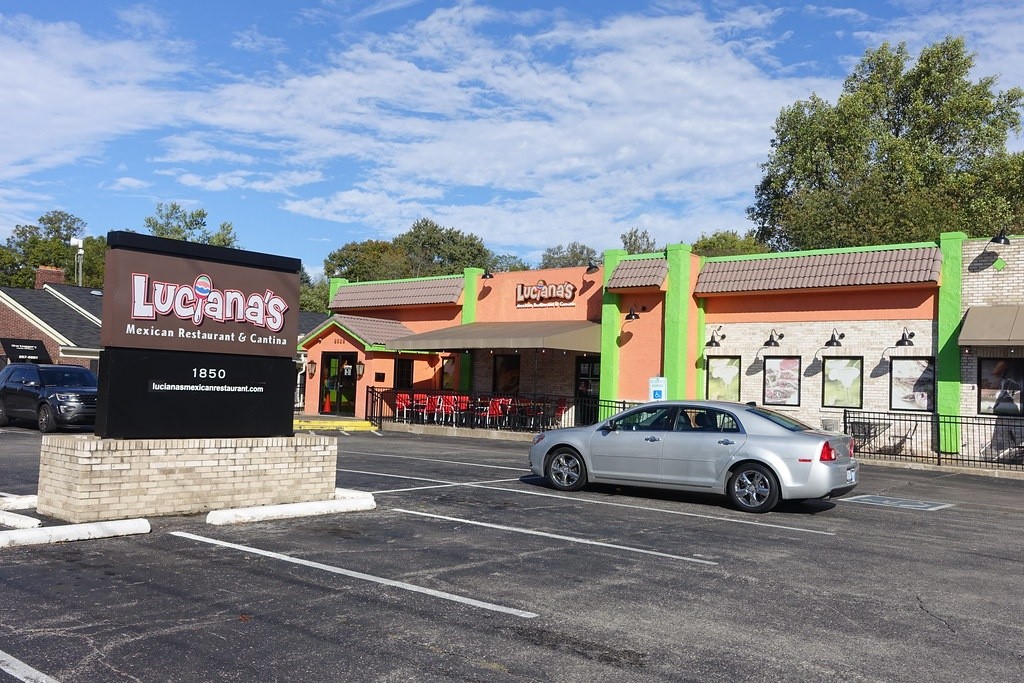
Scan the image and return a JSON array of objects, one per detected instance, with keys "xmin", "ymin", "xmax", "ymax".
[{"xmin": 526, "ymin": 397, "xmax": 861, "ymax": 516}]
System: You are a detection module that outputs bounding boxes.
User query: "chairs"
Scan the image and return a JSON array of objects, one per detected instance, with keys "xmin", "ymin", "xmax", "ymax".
[{"xmin": 393, "ymin": 389, "xmax": 575, "ymax": 432}]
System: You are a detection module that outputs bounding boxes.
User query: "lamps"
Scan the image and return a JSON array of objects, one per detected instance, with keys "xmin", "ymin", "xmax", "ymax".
[
  {"xmin": 307, "ymin": 360, "xmax": 317, "ymax": 374},
  {"xmin": 356, "ymin": 361, "xmax": 365, "ymax": 376},
  {"xmin": 481, "ymin": 268, "xmax": 493, "ymax": 278},
  {"xmin": 625, "ymin": 302, "xmax": 647, "ymax": 319},
  {"xmin": 763, "ymin": 329, "xmax": 784, "ymax": 347},
  {"xmin": 825, "ymin": 328, "xmax": 845, "ymax": 346},
  {"xmin": 582, "ymin": 261, "xmax": 600, "ymax": 282},
  {"xmin": 895, "ymin": 326, "xmax": 915, "ymax": 347},
  {"xmin": 983, "ymin": 223, "xmax": 1011, "ymax": 254},
  {"xmin": 705, "ymin": 329, "xmax": 726, "ymax": 346}
]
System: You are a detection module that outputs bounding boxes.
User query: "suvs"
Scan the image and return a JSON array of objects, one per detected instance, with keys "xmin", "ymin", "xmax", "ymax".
[{"xmin": 0, "ymin": 362, "xmax": 100, "ymax": 433}]
